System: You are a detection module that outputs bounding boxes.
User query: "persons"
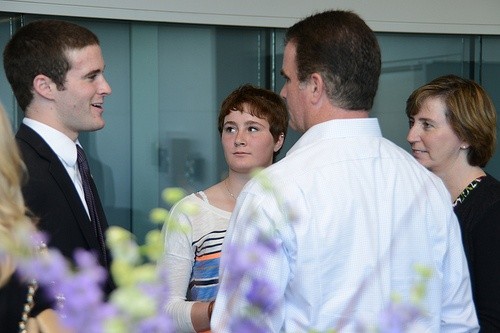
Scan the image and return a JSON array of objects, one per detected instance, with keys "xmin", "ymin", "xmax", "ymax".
[
  {"xmin": 0, "ymin": 18, "xmax": 120, "ymax": 333},
  {"xmin": 405, "ymin": 74, "xmax": 500, "ymax": 332},
  {"xmin": 210, "ymin": 10, "xmax": 481, "ymax": 333},
  {"xmin": 154, "ymin": 83, "xmax": 289, "ymax": 333},
  {"xmin": 1, "ymin": 101, "xmax": 72, "ymax": 333}
]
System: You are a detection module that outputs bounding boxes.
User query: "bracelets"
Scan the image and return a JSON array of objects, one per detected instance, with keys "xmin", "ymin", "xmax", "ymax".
[{"xmin": 207, "ymin": 299, "xmax": 214, "ymax": 320}]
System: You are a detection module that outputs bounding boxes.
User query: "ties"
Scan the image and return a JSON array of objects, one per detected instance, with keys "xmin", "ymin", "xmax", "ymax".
[{"xmin": 75, "ymin": 144, "xmax": 115, "ymax": 291}]
